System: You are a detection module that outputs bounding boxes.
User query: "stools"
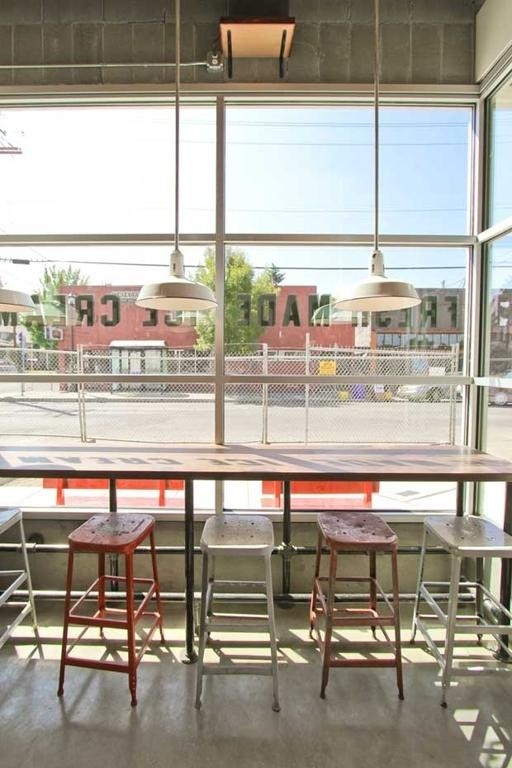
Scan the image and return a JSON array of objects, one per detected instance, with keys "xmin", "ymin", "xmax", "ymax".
[
  {"xmin": 309, "ymin": 514, "xmax": 405, "ymax": 702},
  {"xmin": 410, "ymin": 517, "xmax": 512, "ymax": 708},
  {"xmin": 193, "ymin": 514, "xmax": 283, "ymax": 714},
  {"xmin": 1, "ymin": 509, "xmax": 42, "ymax": 655},
  {"xmin": 56, "ymin": 513, "xmax": 166, "ymax": 710}
]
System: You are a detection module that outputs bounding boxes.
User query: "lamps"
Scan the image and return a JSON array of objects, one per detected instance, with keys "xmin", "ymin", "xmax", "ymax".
[
  {"xmin": 134, "ymin": 1, "xmax": 221, "ymax": 312},
  {"xmin": 328, "ymin": 2, "xmax": 423, "ymax": 311}
]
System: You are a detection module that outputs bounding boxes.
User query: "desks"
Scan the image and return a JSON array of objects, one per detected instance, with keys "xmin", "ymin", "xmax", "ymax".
[{"xmin": 0, "ymin": 445, "xmax": 512, "ymax": 661}]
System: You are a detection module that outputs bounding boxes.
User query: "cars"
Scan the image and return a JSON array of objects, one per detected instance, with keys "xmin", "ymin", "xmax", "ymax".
[{"xmin": 397, "ymin": 369, "xmax": 511, "ymax": 407}]
